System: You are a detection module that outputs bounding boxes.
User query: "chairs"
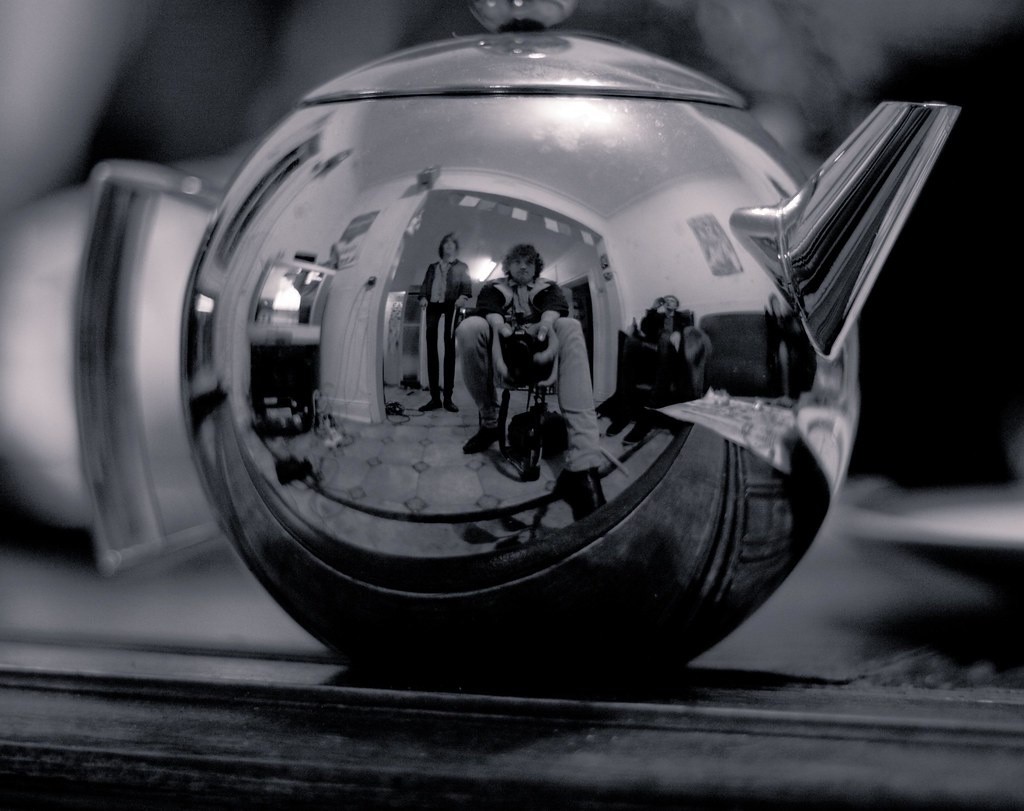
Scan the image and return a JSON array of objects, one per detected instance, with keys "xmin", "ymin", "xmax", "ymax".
[{"xmin": 698, "ymin": 311, "xmax": 786, "ymax": 399}]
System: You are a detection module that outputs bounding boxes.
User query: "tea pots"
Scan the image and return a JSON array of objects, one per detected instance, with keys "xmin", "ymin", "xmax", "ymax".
[{"xmin": 67, "ymin": 1, "xmax": 959, "ymax": 694}]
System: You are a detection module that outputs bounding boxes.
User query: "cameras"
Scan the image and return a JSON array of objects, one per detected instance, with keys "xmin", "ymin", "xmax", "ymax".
[{"xmin": 497, "ymin": 328, "xmax": 555, "ymax": 385}]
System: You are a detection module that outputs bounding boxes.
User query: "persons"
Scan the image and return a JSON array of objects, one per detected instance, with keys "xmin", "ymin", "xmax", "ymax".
[
  {"xmin": 602, "ymin": 294, "xmax": 708, "ymax": 446},
  {"xmin": 417, "ymin": 232, "xmax": 474, "ymax": 413},
  {"xmin": 454, "ymin": 242, "xmax": 609, "ymax": 525}
]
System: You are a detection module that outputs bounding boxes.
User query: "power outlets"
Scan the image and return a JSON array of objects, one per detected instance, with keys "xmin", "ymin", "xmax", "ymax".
[{"xmin": 366, "ymin": 276, "xmax": 376, "ymax": 290}]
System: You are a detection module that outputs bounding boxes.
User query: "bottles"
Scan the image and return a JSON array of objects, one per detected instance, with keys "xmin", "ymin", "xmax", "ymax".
[{"xmin": 522, "ymin": 428, "xmax": 540, "ymax": 480}]
